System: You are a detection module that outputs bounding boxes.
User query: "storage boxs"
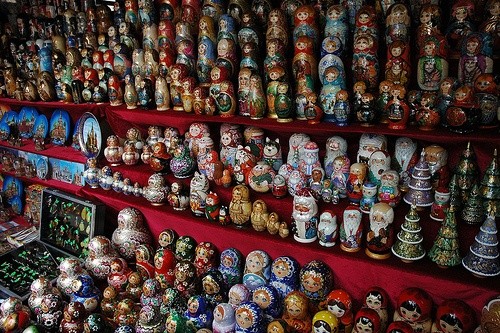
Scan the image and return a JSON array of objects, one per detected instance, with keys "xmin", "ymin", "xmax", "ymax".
[{"xmin": 0, "ymin": 188, "xmax": 105, "ymax": 303}]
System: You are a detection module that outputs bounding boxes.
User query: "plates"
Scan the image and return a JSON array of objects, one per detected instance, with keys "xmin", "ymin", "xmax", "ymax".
[
  {"xmin": 77, "ymin": 111, "xmax": 102, "ymax": 158},
  {"xmin": 36, "ymin": 158, "xmax": 49, "ymax": 179},
  {"xmin": 0, "ymin": 176, "xmax": 22, "ymax": 215},
  {"xmin": 0, "ymin": 103, "xmax": 70, "ymax": 146}
]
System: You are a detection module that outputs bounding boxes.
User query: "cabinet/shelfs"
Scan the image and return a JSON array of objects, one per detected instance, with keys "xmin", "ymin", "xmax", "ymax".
[{"xmin": 0, "ymin": 96, "xmax": 499, "ymax": 333}]
